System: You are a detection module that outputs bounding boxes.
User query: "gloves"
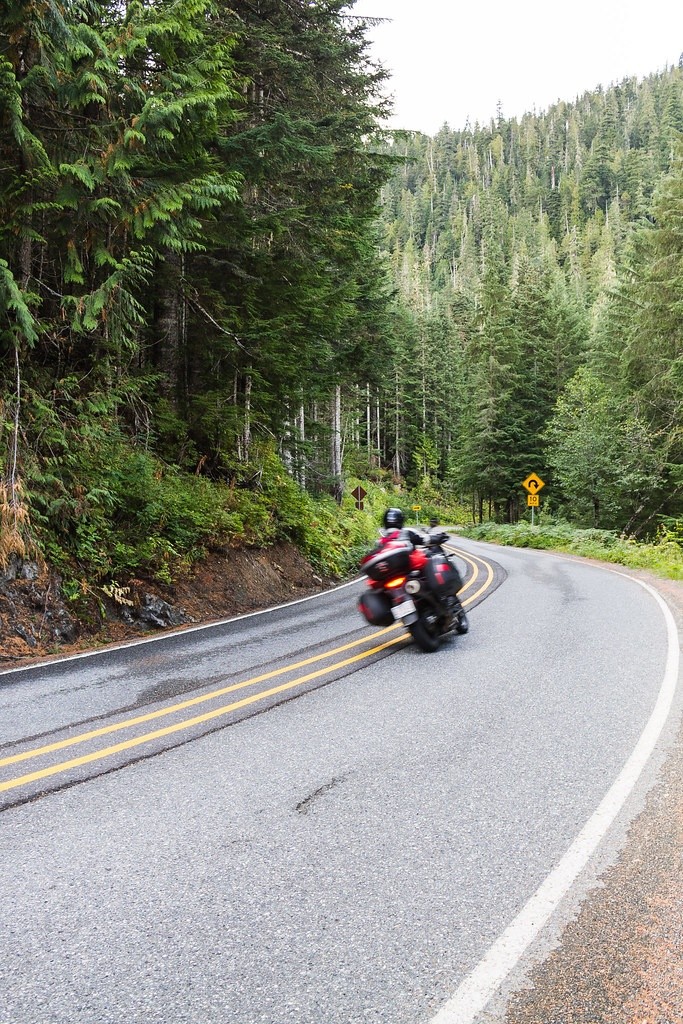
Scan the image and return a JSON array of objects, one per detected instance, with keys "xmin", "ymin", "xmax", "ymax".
[{"xmin": 438, "ymin": 533, "xmax": 449, "ymax": 542}]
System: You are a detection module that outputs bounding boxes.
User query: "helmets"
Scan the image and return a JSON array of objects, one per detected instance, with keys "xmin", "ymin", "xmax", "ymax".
[{"xmin": 383, "ymin": 508, "xmax": 404, "ymax": 527}]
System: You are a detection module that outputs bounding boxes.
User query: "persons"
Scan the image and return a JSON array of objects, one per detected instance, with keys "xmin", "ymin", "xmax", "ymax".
[{"xmin": 367, "ymin": 508, "xmax": 464, "ymax": 617}]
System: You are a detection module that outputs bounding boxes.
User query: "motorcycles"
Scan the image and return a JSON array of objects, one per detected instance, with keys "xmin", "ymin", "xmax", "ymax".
[{"xmin": 355, "ymin": 509, "xmax": 470, "ymax": 655}]
